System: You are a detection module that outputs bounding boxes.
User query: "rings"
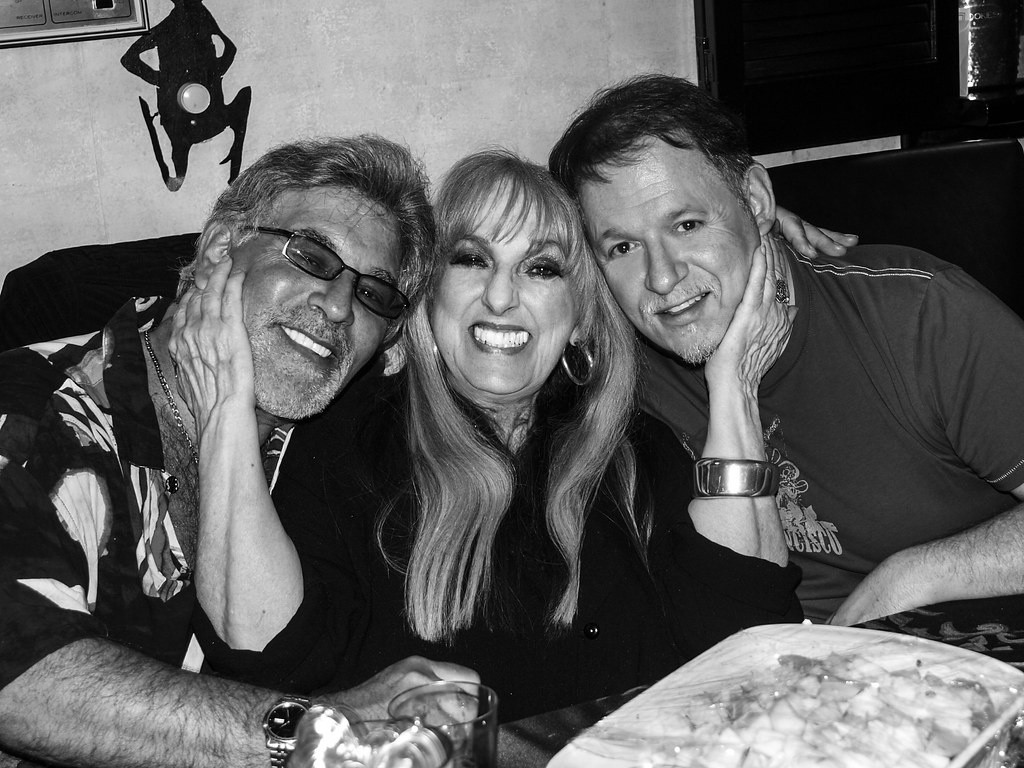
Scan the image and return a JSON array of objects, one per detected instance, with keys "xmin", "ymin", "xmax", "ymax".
[
  {"xmin": 175, "ymin": 276, "xmax": 189, "ymax": 298},
  {"xmin": 776, "ymin": 278, "xmax": 790, "ymax": 302}
]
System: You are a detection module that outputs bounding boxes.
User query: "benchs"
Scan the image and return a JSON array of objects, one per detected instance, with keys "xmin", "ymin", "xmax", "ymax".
[{"xmin": 0, "ymin": 141, "xmax": 1024, "ymax": 351}]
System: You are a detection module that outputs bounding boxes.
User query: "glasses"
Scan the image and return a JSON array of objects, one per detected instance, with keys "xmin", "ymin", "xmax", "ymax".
[{"xmin": 236, "ymin": 223, "xmax": 410, "ymax": 319}]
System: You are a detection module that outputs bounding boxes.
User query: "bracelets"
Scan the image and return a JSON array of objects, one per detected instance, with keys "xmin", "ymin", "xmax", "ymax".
[{"xmin": 693, "ymin": 458, "xmax": 781, "ymax": 498}]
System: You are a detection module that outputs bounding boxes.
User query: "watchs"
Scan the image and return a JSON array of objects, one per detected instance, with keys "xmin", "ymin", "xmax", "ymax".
[{"xmin": 262, "ymin": 695, "xmax": 314, "ymax": 768}]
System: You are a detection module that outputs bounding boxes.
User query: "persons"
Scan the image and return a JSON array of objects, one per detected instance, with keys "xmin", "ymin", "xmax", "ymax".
[
  {"xmin": 0, "ymin": 73, "xmax": 480, "ymax": 768},
  {"xmin": 201, "ymin": 149, "xmax": 812, "ymax": 725},
  {"xmin": 549, "ymin": 73, "xmax": 1024, "ymax": 675}
]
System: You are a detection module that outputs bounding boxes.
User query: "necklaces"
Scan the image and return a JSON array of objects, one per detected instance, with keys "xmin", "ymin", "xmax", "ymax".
[{"xmin": 138, "ymin": 320, "xmax": 201, "ymax": 473}]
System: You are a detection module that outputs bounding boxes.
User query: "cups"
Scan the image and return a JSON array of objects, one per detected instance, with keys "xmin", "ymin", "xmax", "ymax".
[{"xmin": 388, "ymin": 681, "xmax": 498, "ymax": 768}]
